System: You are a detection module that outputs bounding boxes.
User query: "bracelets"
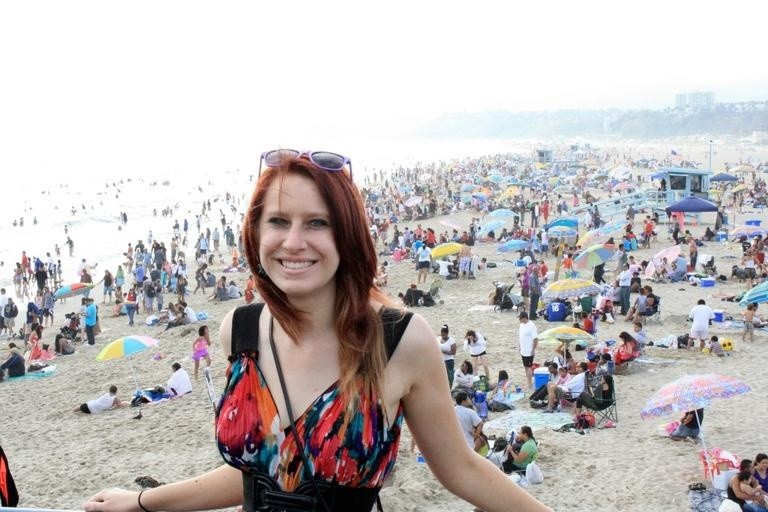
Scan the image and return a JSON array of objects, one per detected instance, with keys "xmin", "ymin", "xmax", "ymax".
[{"xmin": 138, "ymin": 488, "xmax": 149, "ymax": 511}]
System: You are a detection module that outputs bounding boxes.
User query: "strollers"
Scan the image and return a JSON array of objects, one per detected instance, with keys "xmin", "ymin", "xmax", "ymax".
[
  {"xmin": 492, "ymin": 280, "xmax": 525, "ymax": 314},
  {"xmin": 633, "ymin": 232, "xmax": 646, "ymax": 249},
  {"xmin": 58, "ymin": 312, "xmax": 82, "ymax": 344}
]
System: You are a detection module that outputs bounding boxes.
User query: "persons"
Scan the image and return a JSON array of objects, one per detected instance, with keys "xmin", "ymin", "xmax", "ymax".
[
  {"xmin": 154, "ymin": 361, "xmax": 192, "ymax": 396},
  {"xmin": 81, "ymin": 150, "xmax": 555, "ymax": 511},
  {"xmin": 363, "ymin": 149, "xmax": 767, "ymax": 512},
  {"xmin": 73, "ymin": 385, "xmax": 130, "ymax": 414},
  {"xmin": 1, "ymin": 165, "xmax": 261, "ymax": 382}
]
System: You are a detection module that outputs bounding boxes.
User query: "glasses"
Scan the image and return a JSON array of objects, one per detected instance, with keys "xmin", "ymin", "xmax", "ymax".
[{"xmin": 259, "ymin": 149, "xmax": 353, "ymax": 183}]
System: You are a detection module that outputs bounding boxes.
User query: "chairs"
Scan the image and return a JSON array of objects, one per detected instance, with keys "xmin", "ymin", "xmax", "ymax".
[
  {"xmin": 512, "ymin": 439, "xmax": 541, "ymax": 477},
  {"xmin": 564, "ymin": 371, "xmax": 618, "ymax": 425},
  {"xmin": 645, "ymin": 295, "xmax": 663, "ymax": 326}
]
